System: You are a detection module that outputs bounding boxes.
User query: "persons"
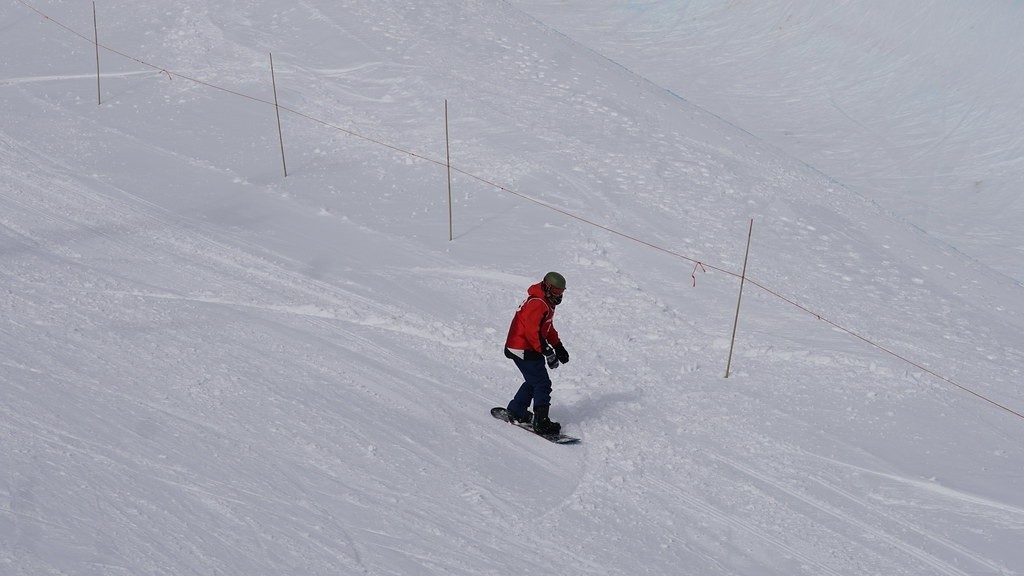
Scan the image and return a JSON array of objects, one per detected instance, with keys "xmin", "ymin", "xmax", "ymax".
[{"xmin": 504, "ymin": 272, "xmax": 570, "ymax": 435}]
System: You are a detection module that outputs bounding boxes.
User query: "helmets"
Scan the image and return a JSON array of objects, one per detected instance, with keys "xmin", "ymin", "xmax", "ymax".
[{"xmin": 544, "ymin": 272, "xmax": 565, "ymax": 290}]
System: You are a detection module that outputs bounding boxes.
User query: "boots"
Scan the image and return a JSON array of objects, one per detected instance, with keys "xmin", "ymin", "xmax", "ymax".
[
  {"xmin": 533, "ymin": 405, "xmax": 561, "ymax": 434},
  {"xmin": 507, "ymin": 399, "xmax": 533, "ymax": 423}
]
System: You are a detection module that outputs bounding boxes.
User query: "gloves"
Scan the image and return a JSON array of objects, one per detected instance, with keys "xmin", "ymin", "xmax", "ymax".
[
  {"xmin": 546, "ymin": 349, "xmax": 559, "ymax": 369},
  {"xmin": 555, "ymin": 343, "xmax": 570, "ymax": 364}
]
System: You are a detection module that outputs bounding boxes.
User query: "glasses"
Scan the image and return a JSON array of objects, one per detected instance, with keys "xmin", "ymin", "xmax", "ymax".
[{"xmin": 549, "ymin": 285, "xmax": 564, "ymax": 297}]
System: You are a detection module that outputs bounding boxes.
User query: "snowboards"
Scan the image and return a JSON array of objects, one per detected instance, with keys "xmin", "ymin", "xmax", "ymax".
[{"xmin": 491, "ymin": 407, "xmax": 581, "ymax": 445}]
